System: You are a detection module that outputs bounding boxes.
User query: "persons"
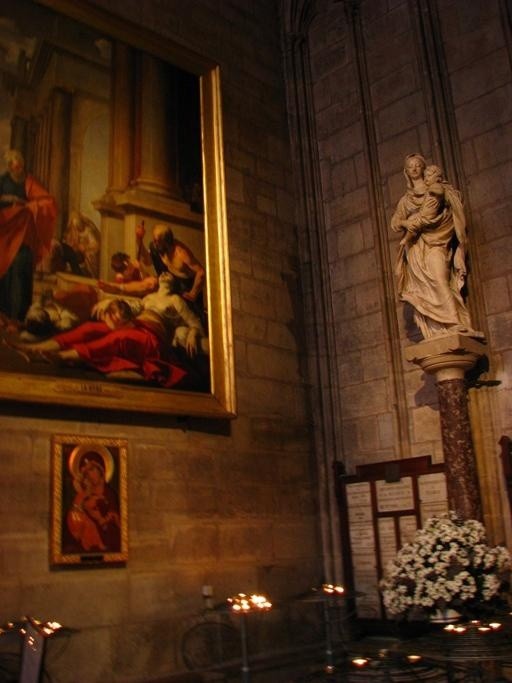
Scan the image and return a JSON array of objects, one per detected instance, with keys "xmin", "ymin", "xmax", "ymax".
[
  {"xmin": 61, "ymin": 443, "xmax": 124, "ymax": 551},
  {"xmin": 397, "ymin": 160, "xmax": 447, "ymax": 246},
  {"xmin": 388, "ymin": 151, "xmax": 481, "ymax": 339}
]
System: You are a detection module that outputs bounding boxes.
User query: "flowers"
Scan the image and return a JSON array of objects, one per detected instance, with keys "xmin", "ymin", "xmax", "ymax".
[{"xmin": 374, "ymin": 507, "xmax": 507, "ymax": 617}]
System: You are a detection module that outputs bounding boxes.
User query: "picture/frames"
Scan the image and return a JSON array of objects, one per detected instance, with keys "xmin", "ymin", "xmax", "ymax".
[
  {"xmin": 48, "ymin": 426, "xmax": 133, "ymax": 571},
  {"xmin": 1, "ymin": 0, "xmax": 243, "ymax": 422}
]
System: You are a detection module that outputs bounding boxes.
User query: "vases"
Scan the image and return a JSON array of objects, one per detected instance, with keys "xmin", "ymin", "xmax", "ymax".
[{"xmin": 430, "ymin": 603, "xmax": 466, "ymax": 626}]
